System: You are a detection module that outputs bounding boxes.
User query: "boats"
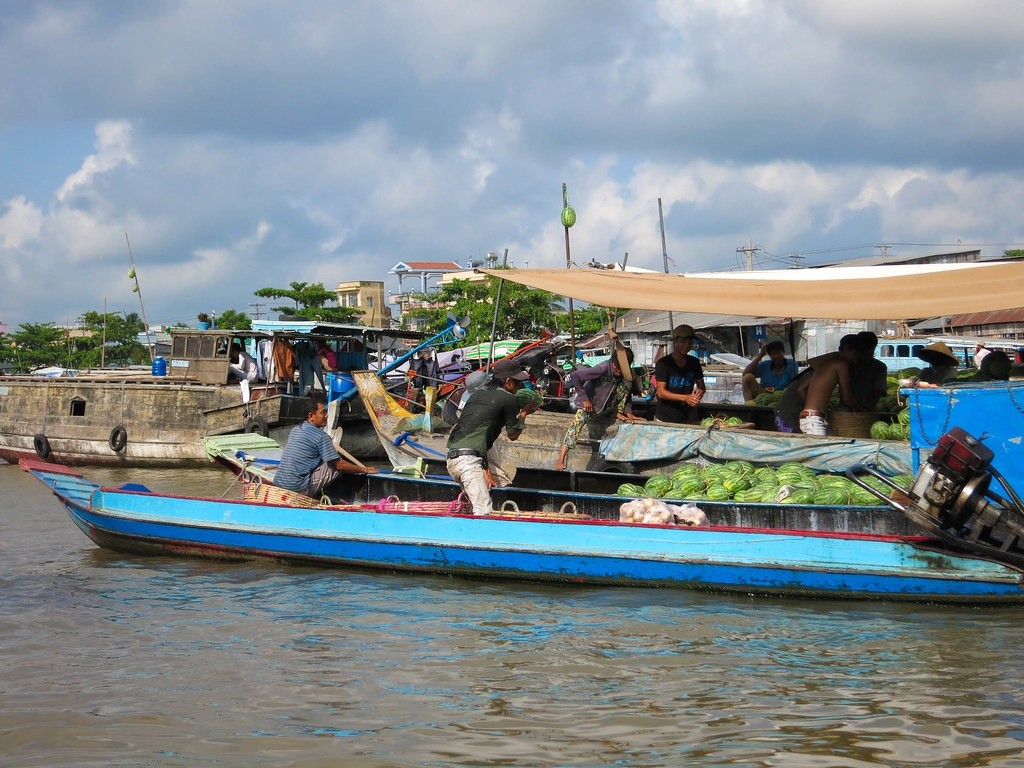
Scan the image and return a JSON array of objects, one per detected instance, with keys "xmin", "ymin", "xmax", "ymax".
[
  {"xmin": 19, "ymin": 363, "xmax": 1024, "ymax": 602},
  {"xmin": 0, "ymin": 328, "xmax": 416, "ymax": 468},
  {"xmin": 872, "ymin": 340, "xmax": 976, "ymax": 372}
]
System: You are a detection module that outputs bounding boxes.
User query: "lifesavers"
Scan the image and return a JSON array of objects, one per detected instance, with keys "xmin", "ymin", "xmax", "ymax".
[
  {"xmin": 109, "ymin": 426, "xmax": 126, "ymax": 452},
  {"xmin": 245, "ymin": 417, "xmax": 269, "ymax": 437},
  {"xmin": 33, "ymin": 434, "xmax": 49, "ymax": 461}
]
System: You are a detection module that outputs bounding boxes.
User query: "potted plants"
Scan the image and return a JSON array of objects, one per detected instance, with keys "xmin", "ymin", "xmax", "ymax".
[{"xmin": 198, "ymin": 313, "xmax": 210, "ymax": 330}]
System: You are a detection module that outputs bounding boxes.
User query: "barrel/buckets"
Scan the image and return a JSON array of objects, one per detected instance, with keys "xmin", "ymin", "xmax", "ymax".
[{"xmin": 333, "ymin": 374, "xmax": 355, "ymax": 400}]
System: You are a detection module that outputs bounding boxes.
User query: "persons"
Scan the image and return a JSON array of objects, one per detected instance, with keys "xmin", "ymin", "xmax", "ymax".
[
  {"xmin": 555, "ymin": 347, "xmax": 647, "ymax": 471},
  {"xmin": 446, "ymin": 360, "xmax": 540, "ymax": 515},
  {"xmin": 839, "ymin": 331, "xmax": 887, "ymax": 412},
  {"xmin": 774, "ymin": 352, "xmax": 840, "ymax": 432},
  {"xmin": 969, "ymin": 351, "xmax": 1011, "ymax": 382},
  {"xmin": 885, "ymin": 345, "xmax": 899, "ymax": 357},
  {"xmin": 974, "ymin": 340, "xmax": 991, "ymax": 370},
  {"xmin": 653, "ymin": 325, "xmax": 706, "ymax": 423},
  {"xmin": 229, "ymin": 343, "xmax": 259, "ymax": 383},
  {"xmin": 272, "ymin": 398, "xmax": 378, "ymax": 498},
  {"xmin": 742, "ymin": 341, "xmax": 799, "ymax": 401},
  {"xmin": 799, "ymin": 334, "xmax": 871, "ymax": 435},
  {"xmin": 919, "ymin": 341, "xmax": 958, "ymax": 383}
]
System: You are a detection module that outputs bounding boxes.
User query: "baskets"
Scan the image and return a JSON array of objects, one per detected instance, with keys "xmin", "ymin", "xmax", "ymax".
[
  {"xmin": 490, "ymin": 500, "xmax": 589, "ymax": 520},
  {"xmin": 244, "ymin": 475, "xmax": 320, "ymax": 507},
  {"xmin": 318, "ymin": 493, "xmax": 473, "ymax": 514}
]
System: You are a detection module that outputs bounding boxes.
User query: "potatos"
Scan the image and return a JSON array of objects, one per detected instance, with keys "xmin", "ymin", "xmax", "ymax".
[{"xmin": 618, "ymin": 497, "xmax": 710, "ymax": 526}]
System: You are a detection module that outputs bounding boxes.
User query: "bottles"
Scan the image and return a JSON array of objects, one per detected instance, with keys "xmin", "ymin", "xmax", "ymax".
[{"xmin": 153, "ymin": 355, "xmax": 166, "ymax": 377}]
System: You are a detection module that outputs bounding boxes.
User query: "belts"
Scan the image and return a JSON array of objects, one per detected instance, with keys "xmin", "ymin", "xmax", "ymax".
[
  {"xmin": 447, "ymin": 450, "xmax": 481, "ymax": 457},
  {"xmin": 800, "ymin": 411, "xmax": 825, "ymax": 418}
]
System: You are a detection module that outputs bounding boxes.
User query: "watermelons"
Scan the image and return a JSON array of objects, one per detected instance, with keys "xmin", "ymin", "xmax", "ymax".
[
  {"xmin": 515, "ymin": 388, "xmax": 542, "ymax": 409},
  {"xmin": 701, "ymin": 416, "xmax": 744, "ymax": 428},
  {"xmin": 560, "ymin": 207, "xmax": 576, "ymax": 227},
  {"xmin": 829, "ymin": 351, "xmax": 1024, "ymax": 442},
  {"xmin": 617, "ymin": 460, "xmax": 914, "ymax": 506},
  {"xmin": 752, "ymin": 391, "xmax": 784, "ymax": 407}
]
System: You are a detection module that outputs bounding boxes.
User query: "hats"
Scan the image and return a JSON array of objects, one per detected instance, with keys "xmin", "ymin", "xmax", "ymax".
[
  {"xmin": 674, "ymin": 325, "xmax": 698, "ymax": 339},
  {"xmin": 977, "ymin": 341, "xmax": 985, "ymax": 346},
  {"xmin": 458, "ymin": 371, "xmax": 491, "ymax": 409},
  {"xmin": 493, "ymin": 360, "xmax": 530, "ymax": 380},
  {"xmin": 917, "ymin": 341, "xmax": 959, "ymax": 366}
]
